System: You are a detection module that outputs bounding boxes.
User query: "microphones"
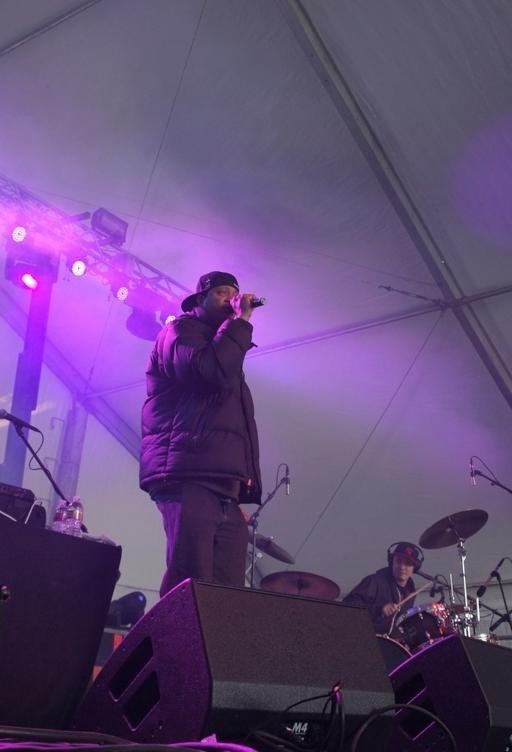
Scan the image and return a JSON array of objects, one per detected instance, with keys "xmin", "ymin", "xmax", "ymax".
[
  {"xmin": 470, "ymin": 461, "xmax": 476, "ymax": 486},
  {"xmin": 226, "ymin": 297, "xmax": 266, "ymax": 314},
  {"xmin": 430, "ymin": 576, "xmax": 438, "ymax": 598},
  {"xmin": 1, "ymin": 408, "xmax": 40, "ymax": 434},
  {"xmin": 476, "ymin": 558, "xmax": 505, "ymax": 597},
  {"xmin": 285, "ymin": 466, "xmax": 291, "ymax": 495}
]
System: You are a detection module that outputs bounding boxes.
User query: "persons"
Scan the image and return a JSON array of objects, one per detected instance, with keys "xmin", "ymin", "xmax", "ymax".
[
  {"xmin": 138, "ymin": 270, "xmax": 267, "ymax": 597},
  {"xmin": 343, "ymin": 541, "xmax": 424, "ymax": 634}
]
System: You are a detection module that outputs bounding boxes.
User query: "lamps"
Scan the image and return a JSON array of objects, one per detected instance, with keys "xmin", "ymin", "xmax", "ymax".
[{"xmin": 0, "ymin": 173, "xmax": 196, "ymax": 341}]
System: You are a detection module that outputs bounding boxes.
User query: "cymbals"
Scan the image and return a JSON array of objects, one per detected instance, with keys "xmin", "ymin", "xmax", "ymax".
[
  {"xmin": 258, "ymin": 570, "xmax": 340, "ymax": 601},
  {"xmin": 421, "ymin": 510, "xmax": 489, "ymax": 549},
  {"xmin": 249, "ymin": 527, "xmax": 295, "ymax": 565}
]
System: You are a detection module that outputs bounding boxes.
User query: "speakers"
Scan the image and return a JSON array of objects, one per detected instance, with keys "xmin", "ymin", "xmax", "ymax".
[
  {"xmin": 70, "ymin": 576, "xmax": 396, "ymax": 742},
  {"xmin": 388, "ymin": 631, "xmax": 511, "ymax": 750}
]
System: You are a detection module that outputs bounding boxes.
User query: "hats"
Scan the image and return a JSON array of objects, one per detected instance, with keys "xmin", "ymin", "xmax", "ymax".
[
  {"xmin": 181, "ymin": 272, "xmax": 239, "ymax": 312},
  {"xmin": 394, "ymin": 543, "xmax": 419, "ymax": 563}
]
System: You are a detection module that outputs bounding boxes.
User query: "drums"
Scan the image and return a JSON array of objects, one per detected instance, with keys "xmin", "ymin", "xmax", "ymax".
[
  {"xmin": 395, "ymin": 602, "xmax": 454, "ymax": 654},
  {"xmin": 470, "ymin": 634, "xmax": 496, "ymax": 645}
]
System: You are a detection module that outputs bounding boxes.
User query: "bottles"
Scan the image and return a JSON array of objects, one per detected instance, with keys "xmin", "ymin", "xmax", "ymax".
[{"xmin": 51, "ymin": 496, "xmax": 83, "ymax": 538}]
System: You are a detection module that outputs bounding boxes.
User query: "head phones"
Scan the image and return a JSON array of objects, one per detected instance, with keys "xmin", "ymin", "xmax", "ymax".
[{"xmin": 386, "ymin": 541, "xmax": 424, "ymax": 574}]
